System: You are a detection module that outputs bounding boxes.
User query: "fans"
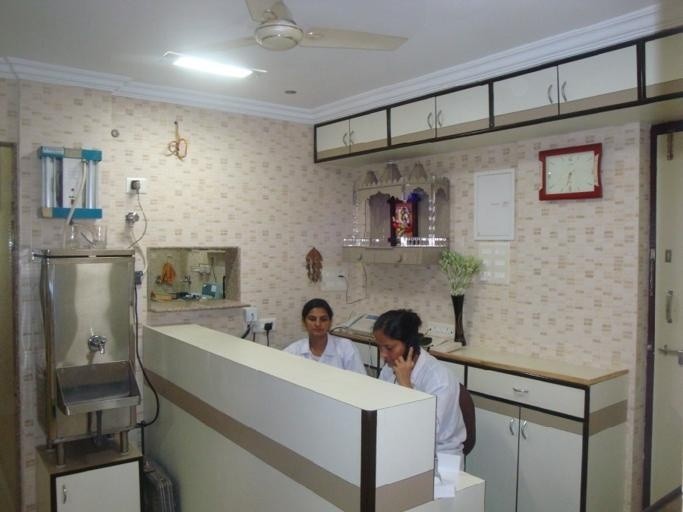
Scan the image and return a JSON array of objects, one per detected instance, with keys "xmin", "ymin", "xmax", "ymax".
[{"xmin": 180, "ymin": 0, "xmax": 409, "ymax": 52}]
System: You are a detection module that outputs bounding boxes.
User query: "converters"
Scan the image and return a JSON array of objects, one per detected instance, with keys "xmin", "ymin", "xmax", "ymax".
[{"xmin": 264, "ymin": 322, "xmax": 272, "ymax": 332}]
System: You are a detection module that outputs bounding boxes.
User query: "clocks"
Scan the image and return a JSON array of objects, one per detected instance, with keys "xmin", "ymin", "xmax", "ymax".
[{"xmin": 538, "ymin": 142, "xmax": 604, "ymax": 201}]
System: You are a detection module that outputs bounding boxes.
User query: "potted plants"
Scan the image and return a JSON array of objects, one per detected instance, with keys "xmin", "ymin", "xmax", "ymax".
[{"xmin": 437, "ymin": 246, "xmax": 481, "ymax": 346}]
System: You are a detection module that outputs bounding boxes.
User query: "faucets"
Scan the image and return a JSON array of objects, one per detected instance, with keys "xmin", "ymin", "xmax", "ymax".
[{"xmin": 88, "ymin": 336, "xmax": 106, "ymax": 354}]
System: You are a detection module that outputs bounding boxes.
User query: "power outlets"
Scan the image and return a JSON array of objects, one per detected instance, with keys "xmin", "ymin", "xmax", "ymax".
[
  {"xmin": 126, "ymin": 177, "xmax": 148, "ymax": 194},
  {"xmin": 252, "ymin": 317, "xmax": 276, "ymax": 333}
]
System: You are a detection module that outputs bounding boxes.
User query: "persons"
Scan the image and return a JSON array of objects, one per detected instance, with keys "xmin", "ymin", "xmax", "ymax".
[
  {"xmin": 372, "ymin": 307, "xmax": 467, "ymax": 471},
  {"xmin": 280, "ymin": 297, "xmax": 366, "ymax": 375},
  {"xmin": 396, "ymin": 207, "xmax": 409, "ymax": 238}
]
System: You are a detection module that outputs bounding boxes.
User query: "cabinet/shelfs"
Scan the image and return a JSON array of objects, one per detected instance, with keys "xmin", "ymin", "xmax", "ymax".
[
  {"xmin": 641, "ymin": 25, "xmax": 682, "ymax": 122},
  {"xmin": 35, "ymin": 435, "xmax": 144, "ymax": 512},
  {"xmin": 314, "ymin": 105, "xmax": 389, "ymax": 168},
  {"xmin": 389, "ymin": 79, "xmax": 491, "ymax": 163},
  {"xmin": 352, "ymin": 340, "xmax": 465, "ymax": 400},
  {"xmin": 491, "ymin": 38, "xmax": 642, "ymax": 147},
  {"xmin": 465, "ymin": 366, "xmax": 628, "ymax": 511}
]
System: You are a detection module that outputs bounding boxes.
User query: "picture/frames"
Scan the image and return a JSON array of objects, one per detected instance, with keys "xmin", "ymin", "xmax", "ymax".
[
  {"xmin": 473, "ymin": 167, "xmax": 517, "ymax": 241},
  {"xmin": 387, "ymin": 192, "xmax": 421, "ymax": 248}
]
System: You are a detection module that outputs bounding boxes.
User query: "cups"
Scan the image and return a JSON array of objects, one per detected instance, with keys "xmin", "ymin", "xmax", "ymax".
[
  {"xmin": 92, "ymin": 225, "xmax": 106, "ymax": 249},
  {"xmin": 66, "ymin": 225, "xmax": 80, "ymax": 250}
]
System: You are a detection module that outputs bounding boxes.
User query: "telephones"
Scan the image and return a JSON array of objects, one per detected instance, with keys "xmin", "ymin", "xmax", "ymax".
[
  {"xmin": 344, "ymin": 311, "xmax": 380, "ymax": 334},
  {"xmin": 429, "ymin": 338, "xmax": 462, "ymax": 353},
  {"xmin": 403, "ymin": 334, "xmax": 423, "ymax": 368}
]
